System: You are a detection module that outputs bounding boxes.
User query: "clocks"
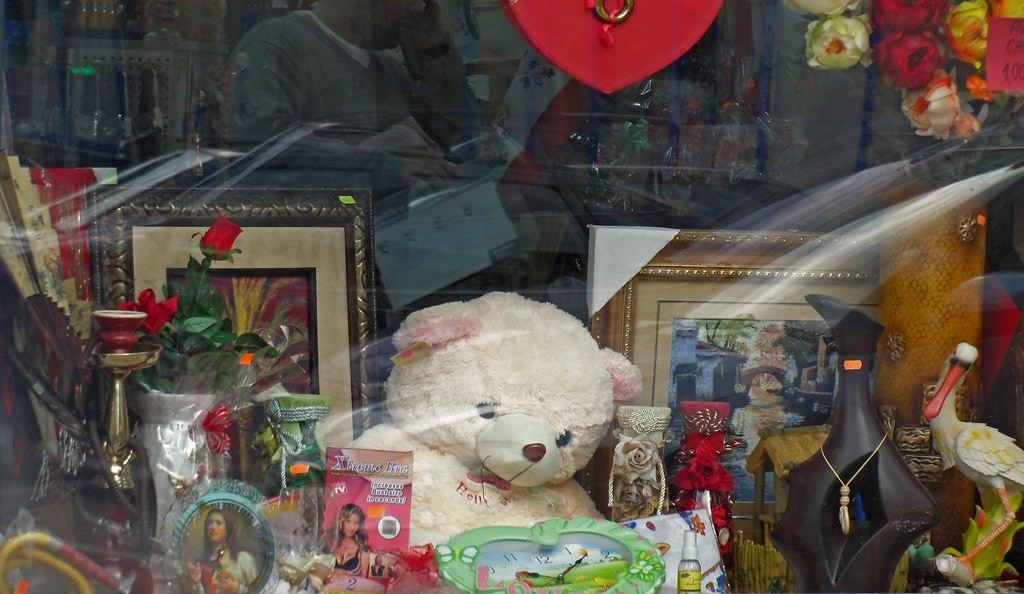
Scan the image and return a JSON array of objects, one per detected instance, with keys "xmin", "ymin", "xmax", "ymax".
[{"xmin": 431, "ymin": 516, "xmax": 666, "ymax": 594}]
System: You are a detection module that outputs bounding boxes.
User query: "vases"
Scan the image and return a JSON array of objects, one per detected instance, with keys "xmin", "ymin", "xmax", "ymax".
[{"xmin": 126, "ymin": 391, "xmax": 229, "ymax": 553}]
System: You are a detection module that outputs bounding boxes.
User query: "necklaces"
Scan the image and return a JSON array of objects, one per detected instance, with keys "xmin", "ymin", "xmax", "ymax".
[{"xmin": 820, "ymin": 431, "xmax": 888, "ymax": 535}]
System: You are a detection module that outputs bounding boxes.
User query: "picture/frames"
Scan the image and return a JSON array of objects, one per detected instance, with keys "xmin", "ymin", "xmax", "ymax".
[
  {"xmin": 586, "ymin": 221, "xmax": 880, "ymax": 547},
  {"xmin": 88, "ymin": 183, "xmax": 378, "ymax": 496}
]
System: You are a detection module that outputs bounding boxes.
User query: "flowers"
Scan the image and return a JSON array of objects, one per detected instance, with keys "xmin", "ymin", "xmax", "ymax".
[
  {"xmin": 114, "ymin": 211, "xmax": 287, "ymax": 399},
  {"xmin": 775, "ymin": 1, "xmax": 1024, "ymax": 141}
]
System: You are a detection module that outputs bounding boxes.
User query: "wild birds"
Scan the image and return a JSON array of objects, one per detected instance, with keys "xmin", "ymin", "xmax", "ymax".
[{"xmin": 925, "ymin": 341, "xmax": 1024, "ymax": 586}]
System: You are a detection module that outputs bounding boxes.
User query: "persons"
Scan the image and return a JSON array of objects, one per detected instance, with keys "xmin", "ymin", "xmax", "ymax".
[
  {"xmin": 185, "ymin": 509, "xmax": 259, "ymax": 594},
  {"xmin": 370, "ymin": 555, "xmax": 388, "ymax": 577},
  {"xmin": 323, "ymin": 503, "xmax": 369, "ymax": 578},
  {"xmin": 226, "ymin": 0, "xmax": 540, "ymax": 302}
]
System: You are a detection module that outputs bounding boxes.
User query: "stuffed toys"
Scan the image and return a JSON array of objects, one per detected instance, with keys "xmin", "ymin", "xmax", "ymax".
[{"xmin": 348, "ymin": 290, "xmax": 644, "ymax": 550}]
